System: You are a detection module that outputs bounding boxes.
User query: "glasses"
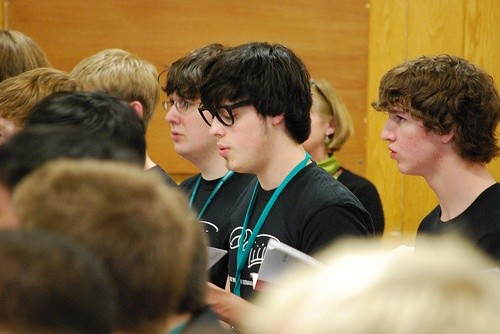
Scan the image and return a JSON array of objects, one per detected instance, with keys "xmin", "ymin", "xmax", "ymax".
[
  {"xmin": 158, "ymin": 96, "xmax": 200, "ymax": 112},
  {"xmin": 198, "ymin": 99, "xmax": 253, "ymax": 127}
]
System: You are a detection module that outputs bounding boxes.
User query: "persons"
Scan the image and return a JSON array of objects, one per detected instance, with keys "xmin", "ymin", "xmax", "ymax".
[
  {"xmin": 0, "ymin": 27, "xmax": 500, "ymax": 334},
  {"xmin": 371, "ymin": 54, "xmax": 500, "ymax": 267},
  {"xmin": 198, "ymin": 42, "xmax": 371, "ymax": 334},
  {"xmin": 301, "ymin": 77, "xmax": 384, "ymax": 240}
]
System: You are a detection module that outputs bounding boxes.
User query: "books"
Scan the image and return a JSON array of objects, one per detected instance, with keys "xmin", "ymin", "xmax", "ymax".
[{"xmin": 249, "ymin": 239, "xmax": 325, "ymax": 295}]
